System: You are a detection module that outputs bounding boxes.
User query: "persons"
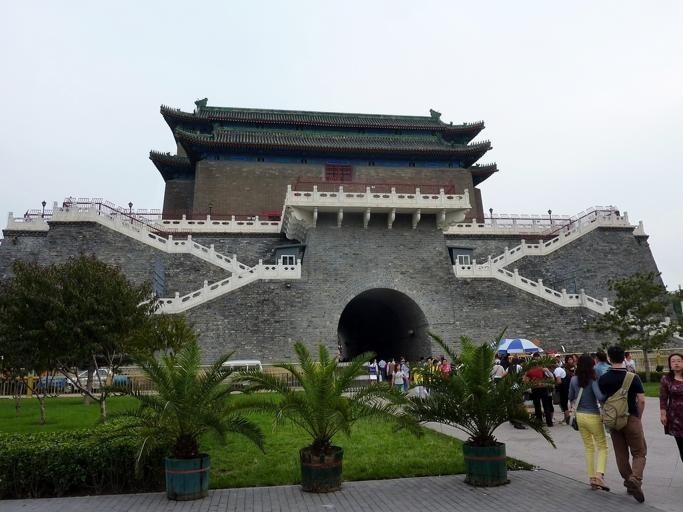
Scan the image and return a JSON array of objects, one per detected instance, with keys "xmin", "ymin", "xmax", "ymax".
[
  {"xmin": 568, "ymin": 354, "xmax": 614, "ymax": 493},
  {"xmin": 488, "ymin": 345, "xmax": 638, "ymax": 430},
  {"xmin": 597, "ymin": 345, "xmax": 647, "ymax": 503},
  {"xmin": 658, "ymin": 353, "xmax": 683, "ymax": 461},
  {"xmin": 370, "ymin": 354, "xmax": 464, "ymax": 395}
]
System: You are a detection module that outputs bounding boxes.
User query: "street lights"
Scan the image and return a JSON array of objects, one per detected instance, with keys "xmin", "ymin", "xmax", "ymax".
[
  {"xmin": 207, "ymin": 201, "xmax": 212, "ymax": 216},
  {"xmin": 547, "ymin": 208, "xmax": 553, "ymax": 225},
  {"xmin": 41, "ymin": 200, "xmax": 47, "ymax": 219},
  {"xmin": 489, "ymin": 207, "xmax": 494, "ymax": 218},
  {"xmin": 127, "ymin": 201, "xmax": 133, "ymax": 212}
]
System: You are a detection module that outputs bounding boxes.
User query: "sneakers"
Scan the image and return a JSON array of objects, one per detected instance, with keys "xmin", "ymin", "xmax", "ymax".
[{"xmin": 623, "ymin": 477, "xmax": 646, "ymax": 504}]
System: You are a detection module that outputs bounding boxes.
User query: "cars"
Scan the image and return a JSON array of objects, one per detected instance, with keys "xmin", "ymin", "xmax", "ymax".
[{"xmin": 62, "ymin": 368, "xmax": 119, "ymax": 394}]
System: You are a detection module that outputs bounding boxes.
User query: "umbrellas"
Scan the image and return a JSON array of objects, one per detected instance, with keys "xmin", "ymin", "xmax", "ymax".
[{"xmin": 485, "ymin": 339, "xmax": 544, "ymax": 355}]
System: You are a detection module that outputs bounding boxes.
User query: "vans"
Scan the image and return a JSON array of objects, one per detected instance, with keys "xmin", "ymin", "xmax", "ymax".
[{"xmin": 214, "ymin": 360, "xmax": 266, "ymax": 389}]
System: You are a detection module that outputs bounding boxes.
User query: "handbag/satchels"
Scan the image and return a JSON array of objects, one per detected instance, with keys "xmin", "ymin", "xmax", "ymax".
[
  {"xmin": 600, "ymin": 371, "xmax": 637, "ymax": 432},
  {"xmin": 565, "ymin": 409, "xmax": 580, "ymax": 431}
]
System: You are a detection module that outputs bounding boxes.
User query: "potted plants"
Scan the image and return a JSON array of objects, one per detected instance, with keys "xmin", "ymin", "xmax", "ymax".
[
  {"xmin": 395, "ymin": 327, "xmax": 559, "ymax": 489},
  {"xmin": 220, "ymin": 340, "xmax": 416, "ymax": 495},
  {"xmin": 91, "ymin": 334, "xmax": 287, "ymax": 501}
]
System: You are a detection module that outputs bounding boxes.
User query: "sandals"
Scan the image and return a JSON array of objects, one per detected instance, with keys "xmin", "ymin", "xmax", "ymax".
[{"xmin": 589, "ymin": 475, "xmax": 610, "ymax": 492}]
